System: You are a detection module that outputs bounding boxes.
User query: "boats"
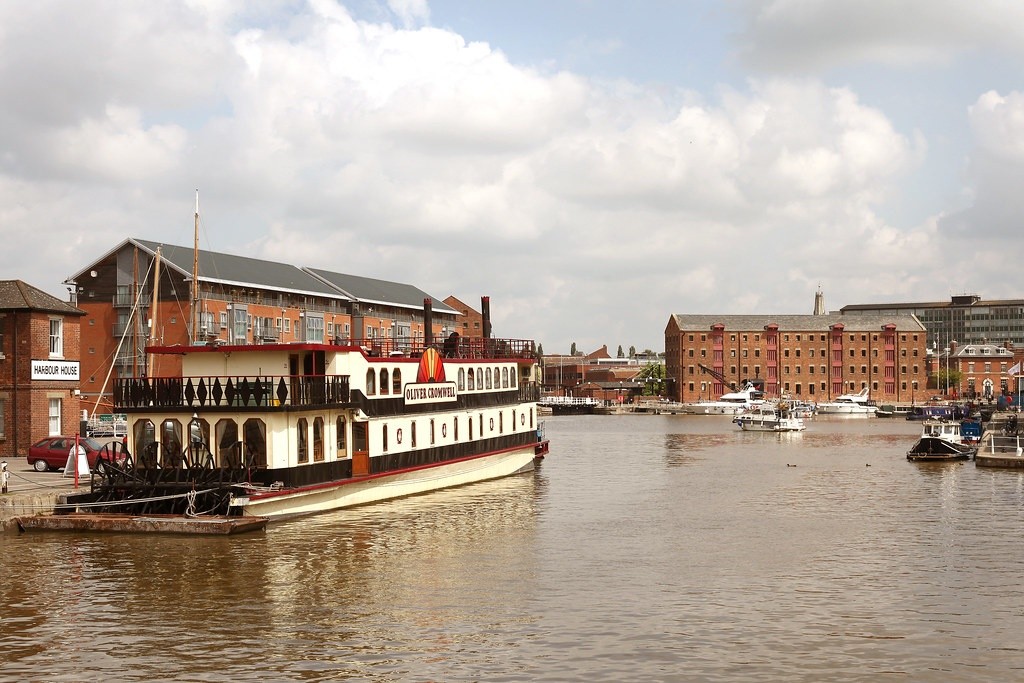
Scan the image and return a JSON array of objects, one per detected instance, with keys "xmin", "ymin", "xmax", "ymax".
[
  {"xmin": 904, "ymin": 417, "xmax": 973, "ymax": 462},
  {"xmin": 813, "ymin": 388, "xmax": 880, "ymax": 414},
  {"xmin": 687, "ymin": 382, "xmax": 776, "ymax": 416},
  {"xmin": 57, "ymin": 188, "xmax": 550, "ymax": 525},
  {"xmin": 732, "ymin": 403, "xmax": 807, "ymax": 432},
  {"xmin": 958, "ymin": 419, "xmax": 986, "ymax": 445},
  {"xmin": 904, "ymin": 405, "xmax": 957, "ymax": 422}
]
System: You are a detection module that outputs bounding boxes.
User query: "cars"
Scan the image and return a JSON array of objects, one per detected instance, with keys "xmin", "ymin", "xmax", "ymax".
[{"xmin": 26, "ymin": 436, "xmax": 127, "ymax": 472}]
